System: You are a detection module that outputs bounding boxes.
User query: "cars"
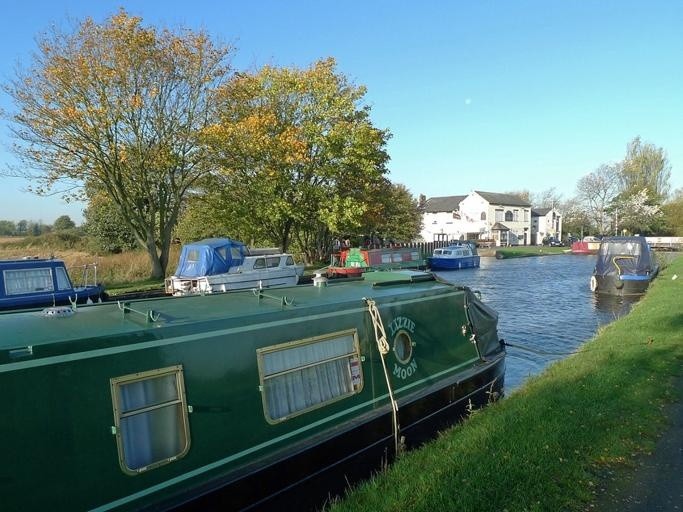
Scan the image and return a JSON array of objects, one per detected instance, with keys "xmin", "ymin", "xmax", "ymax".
[{"xmin": 542, "ymin": 237, "xmax": 565, "ymax": 247}]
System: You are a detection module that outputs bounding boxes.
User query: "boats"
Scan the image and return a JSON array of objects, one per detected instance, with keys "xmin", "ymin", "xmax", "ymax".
[
  {"xmin": 571, "ymin": 235, "xmax": 601, "ymax": 255},
  {"xmin": 591, "ymin": 236, "xmax": 662, "ymax": 297}
]
